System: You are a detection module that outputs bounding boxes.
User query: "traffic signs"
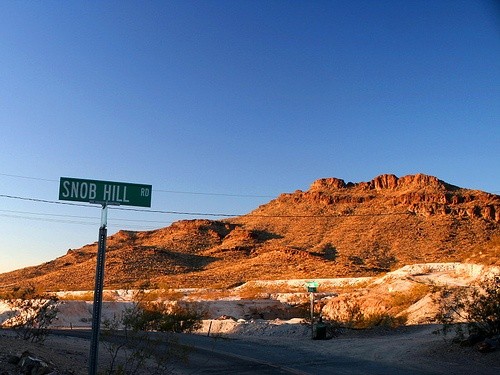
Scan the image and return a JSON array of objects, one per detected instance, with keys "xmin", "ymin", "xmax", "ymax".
[{"xmin": 59, "ymin": 176, "xmax": 152, "ymax": 209}]
